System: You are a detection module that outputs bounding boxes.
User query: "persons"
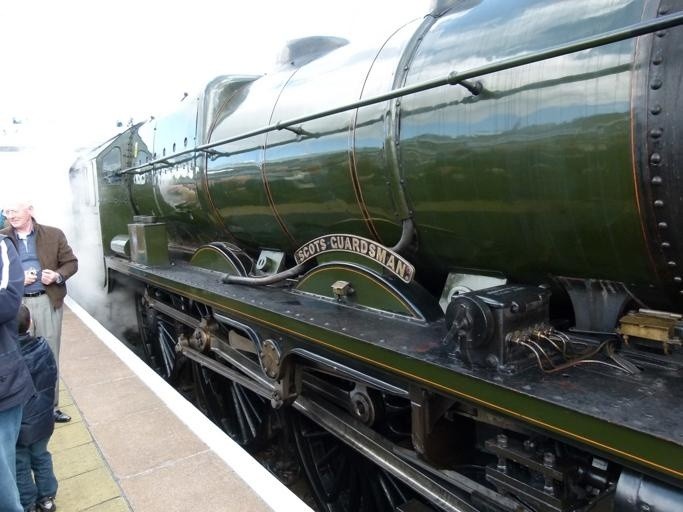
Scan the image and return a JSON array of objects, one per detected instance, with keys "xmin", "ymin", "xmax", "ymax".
[
  {"xmin": 1, "ymin": 200, "xmax": 80, "ymax": 426},
  {"xmin": 11, "ymin": 304, "xmax": 62, "ymax": 512},
  {"xmin": 0, "ymin": 234, "xmax": 32, "ymax": 511}
]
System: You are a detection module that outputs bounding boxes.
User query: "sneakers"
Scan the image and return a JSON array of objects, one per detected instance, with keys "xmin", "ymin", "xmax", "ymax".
[{"xmin": 38, "ymin": 497, "xmax": 56, "ymax": 512}]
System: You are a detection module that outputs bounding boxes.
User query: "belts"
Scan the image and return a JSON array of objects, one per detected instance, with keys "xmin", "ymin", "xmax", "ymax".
[{"xmin": 24, "ymin": 291, "xmax": 45, "ymax": 297}]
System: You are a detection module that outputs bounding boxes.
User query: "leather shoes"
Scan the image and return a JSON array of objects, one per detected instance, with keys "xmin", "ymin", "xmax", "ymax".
[{"xmin": 54, "ymin": 410, "xmax": 71, "ymax": 422}]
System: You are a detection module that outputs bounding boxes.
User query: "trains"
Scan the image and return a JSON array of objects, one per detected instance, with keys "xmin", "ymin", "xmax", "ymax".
[{"xmin": 40, "ymin": 2, "xmax": 683, "ymax": 509}]
